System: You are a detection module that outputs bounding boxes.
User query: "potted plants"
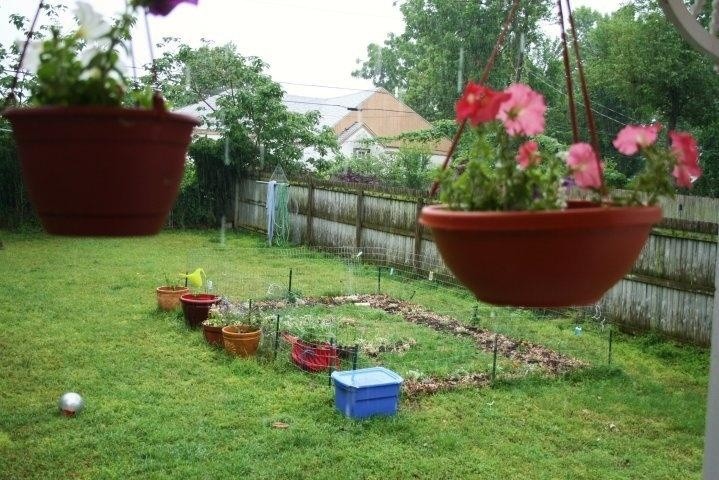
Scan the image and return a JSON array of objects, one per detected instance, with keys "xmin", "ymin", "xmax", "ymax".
[{"xmin": 155, "ymin": 274, "xmax": 189, "ymax": 312}]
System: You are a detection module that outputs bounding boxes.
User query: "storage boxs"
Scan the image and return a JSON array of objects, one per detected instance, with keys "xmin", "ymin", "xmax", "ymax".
[{"xmin": 327, "ymin": 366, "xmax": 403, "ymax": 419}]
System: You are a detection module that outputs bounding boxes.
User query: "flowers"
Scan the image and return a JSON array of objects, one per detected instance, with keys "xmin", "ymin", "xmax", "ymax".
[
  {"xmin": 204, "ymin": 305, "xmax": 259, "ymax": 333},
  {"xmin": 10, "ymin": 0, "xmax": 202, "ymax": 107},
  {"xmin": 184, "ymin": 267, "xmax": 208, "ymax": 300},
  {"xmin": 435, "ymin": 80, "xmax": 701, "ymax": 208}
]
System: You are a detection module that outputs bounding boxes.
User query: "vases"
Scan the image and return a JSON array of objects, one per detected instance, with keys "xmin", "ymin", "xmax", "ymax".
[
  {"xmin": 179, "ymin": 293, "xmax": 221, "ymax": 327},
  {"xmin": 2, "ymin": 108, "xmax": 201, "ymax": 237},
  {"xmin": 418, "ymin": 203, "xmax": 662, "ymax": 308},
  {"xmin": 200, "ymin": 320, "xmax": 260, "ymax": 355}
]
